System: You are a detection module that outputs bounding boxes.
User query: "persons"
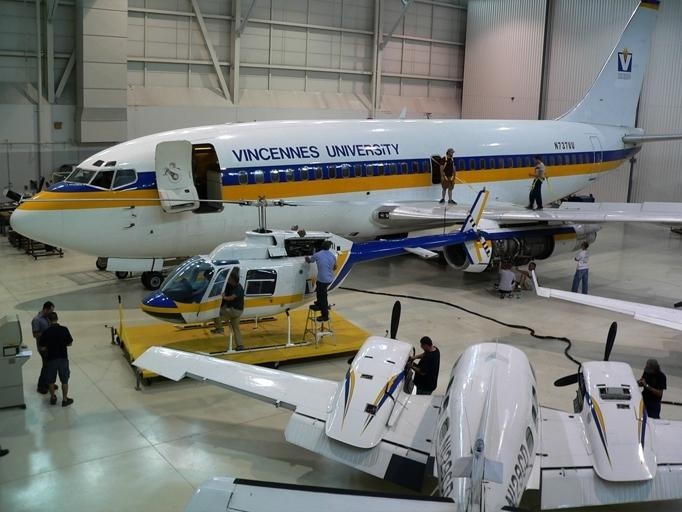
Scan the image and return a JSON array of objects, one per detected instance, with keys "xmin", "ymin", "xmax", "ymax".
[
  {"xmin": 31, "ymin": 300, "xmax": 59, "ymax": 395},
  {"xmin": 39, "ymin": 312, "xmax": 74, "ymax": 407},
  {"xmin": 524, "ymin": 157, "xmax": 548, "ymax": 210},
  {"xmin": 439, "ymin": 149, "xmax": 457, "ymax": 204},
  {"xmin": 515, "ymin": 262, "xmax": 538, "ymax": 292},
  {"xmin": 637, "ymin": 358, "xmax": 667, "ymax": 419},
  {"xmin": 570, "ymin": 241, "xmax": 590, "ymax": 294},
  {"xmin": 210, "ymin": 274, "xmax": 245, "ymax": 352},
  {"xmin": 408, "ymin": 335, "xmax": 441, "ymax": 395},
  {"xmin": 305, "ymin": 241, "xmax": 337, "ymax": 322},
  {"xmin": 496, "ymin": 262, "xmax": 516, "ymax": 297}
]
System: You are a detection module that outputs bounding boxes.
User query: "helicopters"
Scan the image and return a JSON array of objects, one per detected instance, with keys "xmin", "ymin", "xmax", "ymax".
[{"xmin": 19, "ymin": 188, "xmax": 493, "ymax": 357}]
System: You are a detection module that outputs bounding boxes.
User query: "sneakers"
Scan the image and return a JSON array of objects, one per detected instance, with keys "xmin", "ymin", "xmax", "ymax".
[
  {"xmin": 235, "ymin": 345, "xmax": 243, "ymax": 351},
  {"xmin": 509, "ymin": 294, "xmax": 514, "ymax": 298},
  {"xmin": 211, "ymin": 328, "xmax": 224, "ymax": 334},
  {"xmin": 440, "ymin": 198, "xmax": 445, "ymax": 203},
  {"xmin": 448, "ymin": 199, "xmax": 457, "ymax": 205}
]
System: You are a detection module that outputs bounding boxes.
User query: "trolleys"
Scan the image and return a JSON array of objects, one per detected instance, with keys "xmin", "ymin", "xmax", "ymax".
[{"xmin": 8, "ymin": 230, "xmax": 65, "ymax": 260}]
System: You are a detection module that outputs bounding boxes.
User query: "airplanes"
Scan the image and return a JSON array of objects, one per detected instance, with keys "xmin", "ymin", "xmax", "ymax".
[
  {"xmin": 8, "ymin": 0, "xmax": 682, "ymax": 291},
  {"xmin": 133, "ymin": 298, "xmax": 682, "ymax": 512}
]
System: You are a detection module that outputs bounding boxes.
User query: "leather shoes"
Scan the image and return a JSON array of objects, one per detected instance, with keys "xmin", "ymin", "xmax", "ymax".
[
  {"xmin": 317, "ymin": 316, "xmax": 329, "ymax": 321},
  {"xmin": 62, "ymin": 398, "xmax": 72, "ymax": 406},
  {"xmin": 50, "ymin": 395, "xmax": 56, "ymax": 405}
]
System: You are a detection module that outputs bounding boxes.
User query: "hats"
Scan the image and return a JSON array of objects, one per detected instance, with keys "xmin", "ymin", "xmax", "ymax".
[{"xmin": 448, "ymin": 148, "xmax": 454, "ymax": 153}]
